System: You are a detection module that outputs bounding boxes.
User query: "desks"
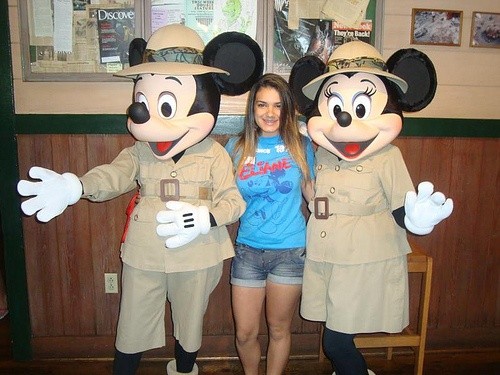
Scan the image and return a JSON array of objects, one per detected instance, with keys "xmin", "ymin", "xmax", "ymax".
[{"xmin": 318, "ymin": 235, "xmax": 434, "ymax": 375}]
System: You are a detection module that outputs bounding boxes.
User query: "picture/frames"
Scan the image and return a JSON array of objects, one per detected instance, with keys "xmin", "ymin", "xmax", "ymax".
[
  {"xmin": 411, "ymin": 8, "xmax": 463, "ymax": 46},
  {"xmin": 267, "ymin": 0, "xmax": 384, "ymax": 83},
  {"xmin": 469, "ymin": 11, "xmax": 500, "ymax": 48},
  {"xmin": 144, "ymin": 0, "xmax": 264, "ymax": 50},
  {"xmin": 16, "ymin": 0, "xmax": 142, "ymax": 82}
]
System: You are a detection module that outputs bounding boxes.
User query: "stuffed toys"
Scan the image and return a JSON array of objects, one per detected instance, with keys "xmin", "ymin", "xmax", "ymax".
[
  {"xmin": 289, "ymin": 39, "xmax": 453, "ymax": 375},
  {"xmin": 17, "ymin": 24, "xmax": 265, "ymax": 375}
]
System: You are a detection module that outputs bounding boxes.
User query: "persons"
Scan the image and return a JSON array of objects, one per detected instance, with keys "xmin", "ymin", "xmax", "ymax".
[
  {"xmin": 118, "ymin": 27, "xmax": 134, "ymax": 70},
  {"xmin": 226, "ymin": 74, "xmax": 316, "ymax": 375}
]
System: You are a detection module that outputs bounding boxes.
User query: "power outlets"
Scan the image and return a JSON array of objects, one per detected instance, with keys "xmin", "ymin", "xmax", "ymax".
[{"xmin": 104, "ymin": 273, "xmax": 117, "ymax": 293}]
